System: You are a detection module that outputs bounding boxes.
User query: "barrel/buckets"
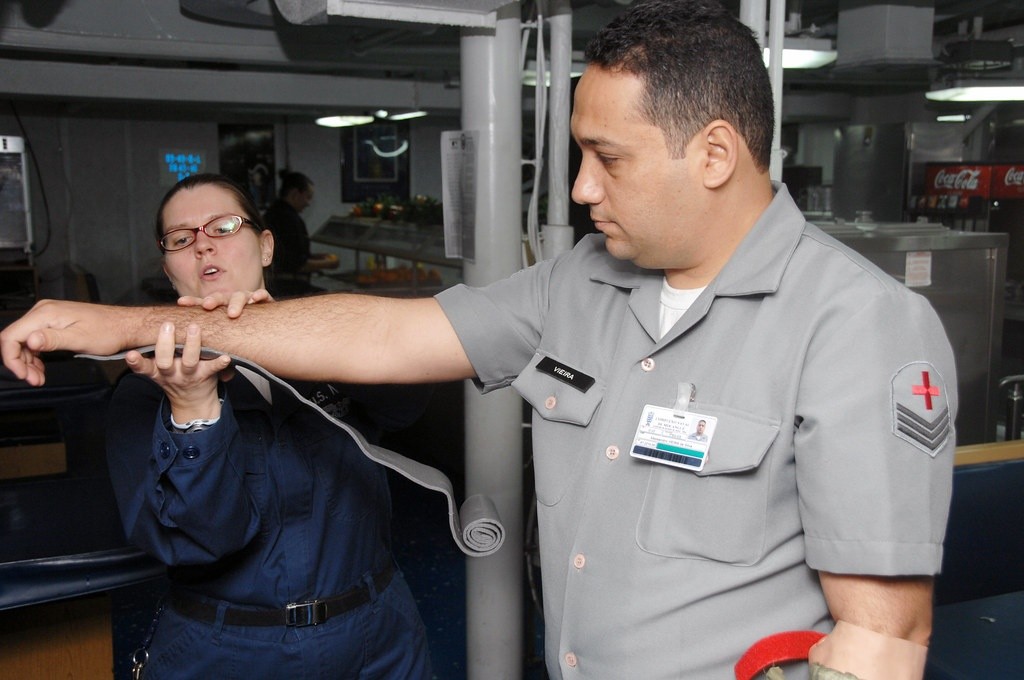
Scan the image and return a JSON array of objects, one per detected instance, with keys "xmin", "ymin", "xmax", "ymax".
[{"xmin": 807, "ymin": 185, "xmax": 834, "ymax": 212}]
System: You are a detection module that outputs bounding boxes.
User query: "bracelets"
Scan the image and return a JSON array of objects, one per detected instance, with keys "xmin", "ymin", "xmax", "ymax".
[{"xmin": 170, "ymin": 398, "xmax": 225, "ymax": 433}]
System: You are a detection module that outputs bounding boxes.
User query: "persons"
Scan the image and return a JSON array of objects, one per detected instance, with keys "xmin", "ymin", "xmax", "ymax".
[
  {"xmin": 0, "ymin": 0, "xmax": 961, "ymax": 680},
  {"xmin": 262, "ymin": 170, "xmax": 341, "ymax": 298},
  {"xmin": 93, "ymin": 174, "xmax": 435, "ymax": 680}
]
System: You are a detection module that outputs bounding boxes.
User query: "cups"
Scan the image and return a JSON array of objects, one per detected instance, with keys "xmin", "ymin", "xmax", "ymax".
[{"xmin": 855, "ymin": 209, "xmax": 879, "ymax": 230}]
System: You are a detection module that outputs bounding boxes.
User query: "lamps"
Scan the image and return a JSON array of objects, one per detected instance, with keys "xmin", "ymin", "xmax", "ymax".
[
  {"xmin": 927, "ymin": 76, "xmax": 1024, "ymax": 102},
  {"xmin": 521, "ymin": 58, "xmax": 587, "ymax": 79},
  {"xmin": 371, "ymin": 123, "xmax": 408, "ymax": 157},
  {"xmin": 763, "ymin": 37, "xmax": 839, "ymax": 73}
]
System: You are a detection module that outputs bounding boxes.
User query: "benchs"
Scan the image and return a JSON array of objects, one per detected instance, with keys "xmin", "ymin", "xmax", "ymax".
[{"xmin": 928, "ymin": 439, "xmax": 1024, "ymax": 680}]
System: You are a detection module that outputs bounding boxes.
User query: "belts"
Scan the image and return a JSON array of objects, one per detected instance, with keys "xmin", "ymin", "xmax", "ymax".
[{"xmin": 169, "ymin": 560, "xmax": 397, "ymax": 627}]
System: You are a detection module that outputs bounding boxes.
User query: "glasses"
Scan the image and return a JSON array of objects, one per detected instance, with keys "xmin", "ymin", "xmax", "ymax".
[{"xmin": 160, "ymin": 215, "xmax": 263, "ymax": 253}]
